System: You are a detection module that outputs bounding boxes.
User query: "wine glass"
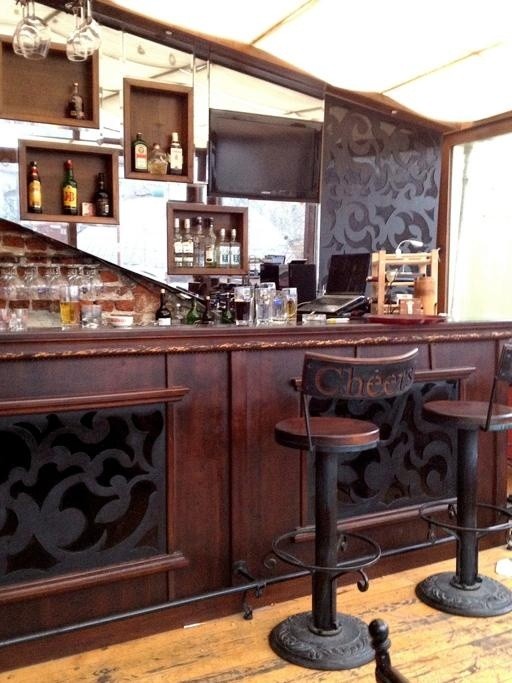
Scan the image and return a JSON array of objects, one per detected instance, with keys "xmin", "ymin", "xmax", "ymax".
[
  {"xmin": 12, "ymin": 1, "xmax": 50, "ymax": 60},
  {"xmin": 0, "ymin": 263, "xmax": 104, "ymax": 300},
  {"xmin": 65, "ymin": 0, "xmax": 102, "ymax": 62}
]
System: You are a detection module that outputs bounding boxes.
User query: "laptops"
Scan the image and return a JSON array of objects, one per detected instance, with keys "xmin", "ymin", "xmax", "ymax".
[{"xmin": 297, "ymin": 254, "xmax": 372, "ymax": 318}]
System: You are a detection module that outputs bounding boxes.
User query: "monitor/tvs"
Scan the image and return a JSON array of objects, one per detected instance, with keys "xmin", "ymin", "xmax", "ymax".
[{"xmin": 205, "ymin": 108, "xmax": 325, "ymax": 203}]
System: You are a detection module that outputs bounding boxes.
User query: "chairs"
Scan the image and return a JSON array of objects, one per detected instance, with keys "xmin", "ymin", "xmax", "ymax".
[
  {"xmin": 268, "ymin": 346, "xmax": 419, "ymax": 671},
  {"xmin": 415, "ymin": 342, "xmax": 512, "ymax": 617}
]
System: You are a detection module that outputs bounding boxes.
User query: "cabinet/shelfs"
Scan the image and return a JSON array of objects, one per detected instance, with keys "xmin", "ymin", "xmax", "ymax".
[{"xmin": 364, "ymin": 251, "xmax": 437, "ymax": 319}]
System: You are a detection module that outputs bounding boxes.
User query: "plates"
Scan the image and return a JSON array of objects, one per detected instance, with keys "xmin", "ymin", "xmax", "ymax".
[{"xmin": 106, "ymin": 316, "xmax": 133, "ymax": 328}]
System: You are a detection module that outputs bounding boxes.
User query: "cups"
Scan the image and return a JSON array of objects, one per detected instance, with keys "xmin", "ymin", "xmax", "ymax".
[
  {"xmin": 255, "ymin": 288, "xmax": 272, "ymax": 328},
  {"xmin": 282, "ymin": 288, "xmax": 297, "ymax": 320},
  {"xmin": 234, "ymin": 286, "xmax": 254, "ymax": 327},
  {"xmin": 59, "ymin": 286, "xmax": 81, "ymax": 326},
  {"xmin": 0, "ymin": 308, "xmax": 9, "ymax": 332},
  {"xmin": 81, "ymin": 305, "xmax": 102, "ymax": 329},
  {"xmin": 259, "ymin": 282, "xmax": 276, "ymax": 297},
  {"xmin": 272, "ymin": 290, "xmax": 288, "ymax": 326},
  {"xmin": 9, "ymin": 308, "xmax": 28, "ymax": 332}
]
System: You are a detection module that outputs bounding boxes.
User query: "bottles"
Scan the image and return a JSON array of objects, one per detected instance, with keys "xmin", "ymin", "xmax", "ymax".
[
  {"xmin": 156, "ymin": 289, "xmax": 171, "ymax": 321},
  {"xmin": 63, "ymin": 159, "xmax": 78, "ymax": 214},
  {"xmin": 221, "ymin": 298, "xmax": 233, "ymax": 323},
  {"xmin": 94, "ymin": 172, "xmax": 110, "ymax": 216},
  {"xmin": 230, "ymin": 229, "xmax": 241, "ymax": 270},
  {"xmin": 183, "ymin": 217, "xmax": 194, "ymax": 268},
  {"xmin": 204, "ymin": 217, "xmax": 217, "ymax": 268},
  {"xmin": 132, "ymin": 132, "xmax": 148, "ymax": 173},
  {"xmin": 173, "ymin": 217, "xmax": 183, "ymax": 268},
  {"xmin": 68, "ymin": 82, "xmax": 85, "ymax": 120},
  {"xmin": 148, "ymin": 142, "xmax": 167, "ymax": 175},
  {"xmin": 216, "ymin": 228, "xmax": 229, "ymax": 268},
  {"xmin": 194, "ymin": 216, "xmax": 205, "ymax": 267},
  {"xmin": 28, "ymin": 160, "xmax": 43, "ymax": 213},
  {"xmin": 187, "ymin": 297, "xmax": 201, "ymax": 323},
  {"xmin": 168, "ymin": 132, "xmax": 183, "ymax": 175},
  {"xmin": 203, "ymin": 296, "xmax": 215, "ymax": 323}
]
